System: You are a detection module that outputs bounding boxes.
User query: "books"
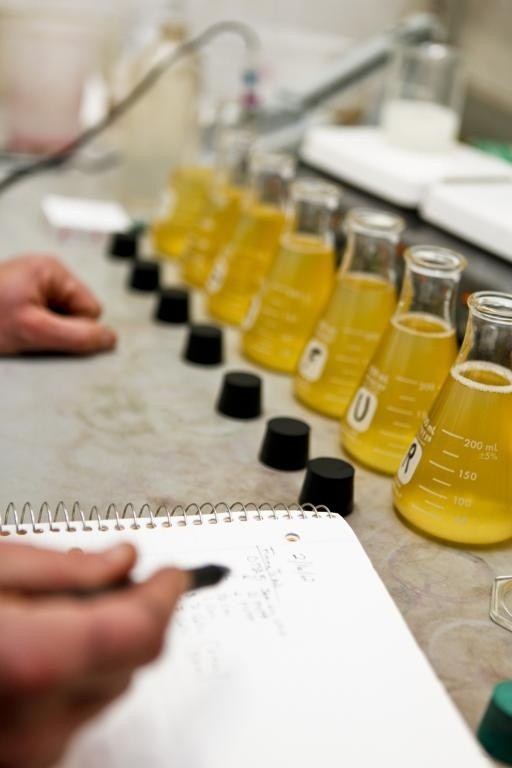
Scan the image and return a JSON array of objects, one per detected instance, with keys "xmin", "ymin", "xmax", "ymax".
[{"xmin": 2, "ymin": 492, "xmax": 495, "ymax": 768}]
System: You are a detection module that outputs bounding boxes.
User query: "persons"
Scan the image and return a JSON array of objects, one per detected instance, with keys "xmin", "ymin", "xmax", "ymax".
[{"xmin": 0, "ymin": 242, "xmax": 193, "ymax": 767}]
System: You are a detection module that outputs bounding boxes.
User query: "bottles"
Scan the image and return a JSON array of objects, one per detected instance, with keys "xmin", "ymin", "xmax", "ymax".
[
  {"xmin": 393, "ymin": 291, "xmax": 512, "ymax": 547},
  {"xmin": 292, "ymin": 206, "xmax": 406, "ymax": 423},
  {"xmin": 199, "ymin": 154, "xmax": 301, "ymax": 337},
  {"xmin": 338, "ymin": 245, "xmax": 467, "ymax": 478},
  {"xmin": 152, "ymin": 143, "xmax": 252, "ymax": 293},
  {"xmin": 240, "ymin": 178, "xmax": 339, "ymax": 370}
]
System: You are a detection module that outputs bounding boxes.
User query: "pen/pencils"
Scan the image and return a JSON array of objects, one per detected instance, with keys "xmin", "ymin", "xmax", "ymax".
[{"xmin": 103, "ymin": 565, "xmax": 230, "ymax": 596}]
